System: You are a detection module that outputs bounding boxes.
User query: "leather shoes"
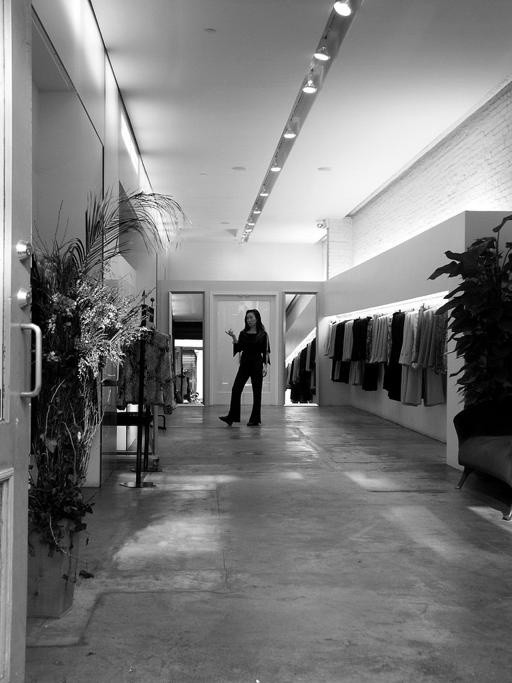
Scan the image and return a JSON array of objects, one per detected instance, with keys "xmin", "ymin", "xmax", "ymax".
[
  {"xmin": 219, "ymin": 416, "xmax": 232, "ymax": 425},
  {"xmin": 247, "ymin": 422, "xmax": 258, "ymax": 426}
]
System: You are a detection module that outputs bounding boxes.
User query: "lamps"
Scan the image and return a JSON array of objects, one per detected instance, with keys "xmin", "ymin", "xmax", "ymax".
[{"xmin": 240, "ymin": 0, "xmax": 362, "ymax": 242}]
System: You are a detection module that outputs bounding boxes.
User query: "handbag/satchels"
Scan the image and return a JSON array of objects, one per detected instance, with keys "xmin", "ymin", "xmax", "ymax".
[{"xmin": 262, "ymin": 335, "xmax": 270, "ymax": 377}]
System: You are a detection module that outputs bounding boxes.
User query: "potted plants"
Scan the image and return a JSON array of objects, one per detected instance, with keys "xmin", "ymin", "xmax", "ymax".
[{"xmin": 27, "ymin": 186, "xmax": 189, "ymax": 620}]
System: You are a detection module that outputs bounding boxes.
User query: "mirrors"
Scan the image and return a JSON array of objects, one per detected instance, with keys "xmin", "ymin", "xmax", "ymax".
[
  {"xmin": 168, "ymin": 290, "xmax": 206, "ymax": 407},
  {"xmin": 282, "ymin": 291, "xmax": 319, "ymax": 407}
]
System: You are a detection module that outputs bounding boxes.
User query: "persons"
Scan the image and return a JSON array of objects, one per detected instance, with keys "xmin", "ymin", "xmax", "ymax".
[{"xmin": 217, "ymin": 308, "xmax": 271, "ymax": 426}]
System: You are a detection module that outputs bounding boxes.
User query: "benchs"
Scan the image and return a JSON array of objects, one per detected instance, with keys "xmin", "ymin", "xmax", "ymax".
[{"xmin": 454, "ymin": 407, "xmax": 512, "ymax": 521}]
